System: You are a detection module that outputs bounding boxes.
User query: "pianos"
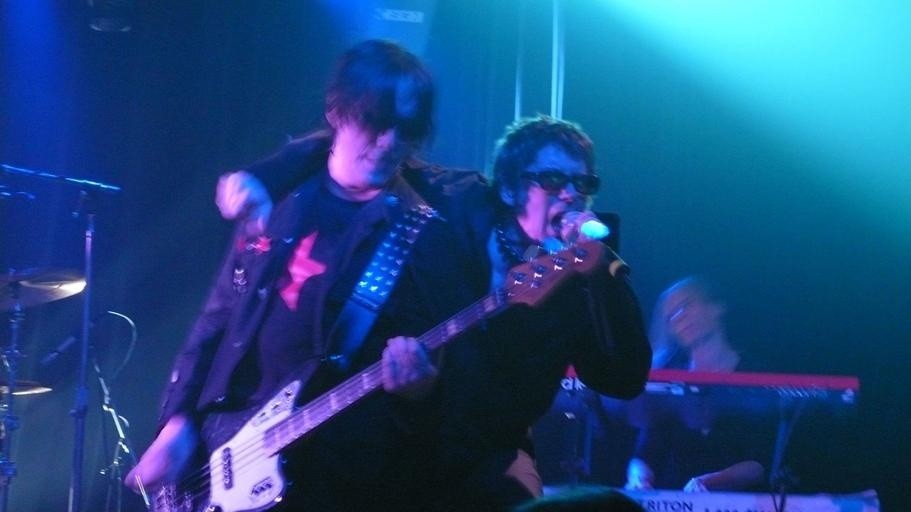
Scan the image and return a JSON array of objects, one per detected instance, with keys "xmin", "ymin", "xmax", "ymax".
[
  {"xmin": 541, "ymin": 488, "xmax": 882, "ymax": 512},
  {"xmin": 558, "ymin": 365, "xmax": 862, "ymax": 412}
]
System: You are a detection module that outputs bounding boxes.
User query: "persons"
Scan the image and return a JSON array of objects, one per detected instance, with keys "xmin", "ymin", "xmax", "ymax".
[
  {"xmin": 215, "ymin": 115, "xmax": 653, "ymax": 510},
  {"xmin": 625, "ymin": 272, "xmax": 775, "ymax": 493},
  {"xmin": 124, "ymin": 39, "xmax": 484, "ymax": 511}
]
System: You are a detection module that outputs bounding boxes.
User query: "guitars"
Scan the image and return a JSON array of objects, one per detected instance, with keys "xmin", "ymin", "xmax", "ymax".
[{"xmin": 150, "ymin": 241, "xmax": 606, "ymax": 512}]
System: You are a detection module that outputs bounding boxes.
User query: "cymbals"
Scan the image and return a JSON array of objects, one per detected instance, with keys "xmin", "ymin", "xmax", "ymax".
[
  {"xmin": 0, "ymin": 379, "xmax": 52, "ymax": 395},
  {"xmin": 0, "ymin": 271, "xmax": 87, "ymax": 315}
]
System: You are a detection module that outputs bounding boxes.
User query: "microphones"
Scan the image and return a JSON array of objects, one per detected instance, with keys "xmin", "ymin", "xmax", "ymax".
[{"xmin": 559, "ymin": 208, "xmax": 633, "ymax": 284}]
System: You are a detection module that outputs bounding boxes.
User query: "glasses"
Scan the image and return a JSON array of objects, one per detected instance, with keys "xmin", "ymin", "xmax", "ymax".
[{"xmin": 522, "ymin": 169, "xmax": 602, "ymax": 197}]
